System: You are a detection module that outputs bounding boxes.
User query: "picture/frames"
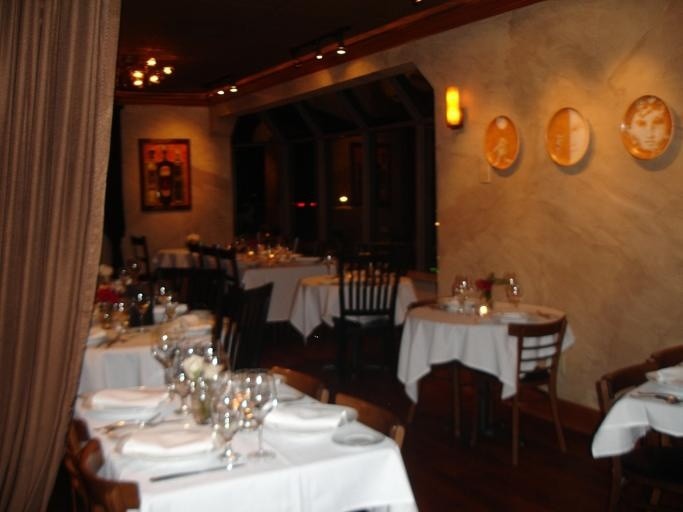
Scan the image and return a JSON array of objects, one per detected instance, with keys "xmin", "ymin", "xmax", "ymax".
[{"xmin": 137, "ymin": 139, "xmax": 192, "ymax": 212}]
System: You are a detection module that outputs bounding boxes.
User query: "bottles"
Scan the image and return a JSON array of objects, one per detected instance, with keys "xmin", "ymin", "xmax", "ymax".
[{"xmin": 146, "ymin": 147, "xmax": 185, "ymax": 206}]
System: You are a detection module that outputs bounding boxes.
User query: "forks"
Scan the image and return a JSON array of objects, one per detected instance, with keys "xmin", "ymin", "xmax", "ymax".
[{"xmin": 631, "ymin": 389, "xmax": 680, "ymax": 409}]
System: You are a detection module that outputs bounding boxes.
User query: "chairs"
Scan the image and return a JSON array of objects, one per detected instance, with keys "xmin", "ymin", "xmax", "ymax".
[
  {"xmin": 645, "ymin": 344, "xmax": 682, "ymax": 378},
  {"xmin": 57, "ymin": 232, "xmax": 417, "ymax": 511},
  {"xmin": 395, "ymin": 296, "xmax": 468, "ymax": 441},
  {"xmin": 470, "ymin": 314, "xmax": 569, "ymax": 467}
]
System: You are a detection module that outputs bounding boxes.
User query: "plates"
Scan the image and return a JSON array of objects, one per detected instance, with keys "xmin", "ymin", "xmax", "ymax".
[
  {"xmin": 481, "ymin": 116, "xmax": 522, "ymax": 173},
  {"xmin": 658, "ymin": 366, "xmax": 683, "ymax": 382},
  {"xmin": 278, "ymin": 385, "xmax": 307, "ymax": 402},
  {"xmin": 333, "ymin": 430, "xmax": 391, "ymax": 449},
  {"xmin": 266, "ymin": 404, "xmax": 358, "ymax": 430},
  {"xmin": 619, "ymin": 94, "xmax": 674, "ymax": 161},
  {"xmin": 541, "ymin": 107, "xmax": 591, "ymax": 168},
  {"xmin": 90, "ymin": 390, "xmax": 157, "ymax": 409},
  {"xmin": 118, "ymin": 425, "xmax": 214, "ymax": 459}
]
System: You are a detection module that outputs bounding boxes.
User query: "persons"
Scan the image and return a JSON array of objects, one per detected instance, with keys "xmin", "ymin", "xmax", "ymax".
[{"xmin": 623, "ymin": 94, "xmax": 671, "ymax": 156}]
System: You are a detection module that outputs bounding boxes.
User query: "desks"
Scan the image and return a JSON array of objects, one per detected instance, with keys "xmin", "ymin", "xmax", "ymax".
[{"xmin": 396, "ymin": 301, "xmax": 577, "ymax": 448}]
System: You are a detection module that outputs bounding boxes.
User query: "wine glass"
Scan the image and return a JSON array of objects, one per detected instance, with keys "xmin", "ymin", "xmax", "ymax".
[
  {"xmin": 154, "ymin": 324, "xmax": 288, "ymax": 465},
  {"xmin": 321, "ymin": 253, "xmax": 395, "ymax": 283},
  {"xmin": 205, "ymin": 235, "xmax": 310, "ymax": 266},
  {"xmin": 448, "ymin": 272, "xmax": 523, "ymax": 316},
  {"xmin": 96, "ymin": 266, "xmax": 174, "ymax": 340}
]
